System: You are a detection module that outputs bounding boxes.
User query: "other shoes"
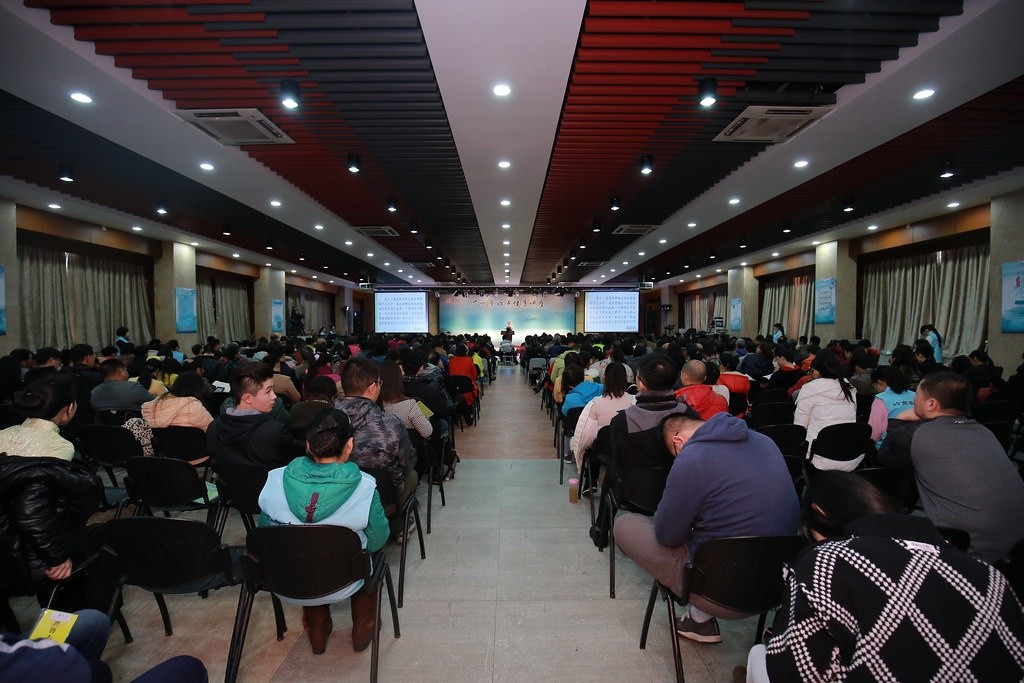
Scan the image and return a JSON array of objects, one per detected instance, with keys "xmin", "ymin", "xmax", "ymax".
[
  {"xmin": 582, "ymin": 479, "xmax": 598, "ymax": 495},
  {"xmin": 589, "ymin": 524, "xmax": 607, "ymax": 547},
  {"xmin": 733, "ymin": 666, "xmax": 746, "ymax": 683},
  {"xmin": 564, "ymin": 454, "xmax": 572, "ymax": 463}
]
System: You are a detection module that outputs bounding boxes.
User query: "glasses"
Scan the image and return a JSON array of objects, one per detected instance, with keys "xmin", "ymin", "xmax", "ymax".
[{"xmin": 367, "ymin": 381, "xmax": 383, "ymax": 387}]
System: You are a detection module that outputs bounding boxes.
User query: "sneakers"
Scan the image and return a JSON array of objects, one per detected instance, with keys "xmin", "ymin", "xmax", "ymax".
[
  {"xmin": 676, "ymin": 617, "xmax": 722, "ymax": 643},
  {"xmin": 395, "ymin": 516, "xmax": 415, "ymax": 544}
]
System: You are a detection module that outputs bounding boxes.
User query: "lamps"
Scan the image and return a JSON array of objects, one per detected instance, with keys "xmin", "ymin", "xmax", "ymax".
[
  {"xmin": 570, "ymin": 251, "xmax": 577, "ymax": 261},
  {"xmin": 592, "ymin": 220, "xmax": 601, "ymax": 233},
  {"xmin": 610, "ymin": 196, "xmax": 620, "ymax": 211},
  {"xmin": 557, "ymin": 265, "xmax": 563, "ymax": 275},
  {"xmin": 666, "ymin": 250, "xmax": 716, "ymax": 275},
  {"xmin": 697, "ymin": 77, "xmax": 718, "ymax": 111},
  {"xmin": 937, "ymin": 158, "xmax": 956, "ymax": 179},
  {"xmin": 739, "ymin": 236, "xmax": 747, "ymax": 249},
  {"xmin": 222, "ymin": 223, "xmax": 366, "ymax": 282},
  {"xmin": 410, "ymin": 220, "xmax": 443, "ymax": 260},
  {"xmin": 445, "ymin": 257, "xmax": 466, "ymax": 285},
  {"xmin": 579, "ymin": 239, "xmax": 587, "ymax": 249},
  {"xmin": 546, "ymin": 278, "xmax": 551, "ymax": 285},
  {"xmin": 504, "ymin": 272, "xmax": 510, "ymax": 278},
  {"xmin": 563, "ymin": 260, "xmax": 569, "ymax": 269},
  {"xmin": 552, "ymin": 273, "xmax": 556, "ymax": 281},
  {"xmin": 640, "ymin": 155, "xmax": 653, "ymax": 177},
  {"xmin": 782, "ymin": 218, "xmax": 792, "ymax": 234},
  {"xmin": 648, "ymin": 273, "xmax": 656, "ymax": 281},
  {"xmin": 276, "ymin": 80, "xmax": 398, "ymax": 214}
]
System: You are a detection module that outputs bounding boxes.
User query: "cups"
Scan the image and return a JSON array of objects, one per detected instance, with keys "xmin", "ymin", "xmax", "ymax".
[{"xmin": 569, "ymin": 478, "xmax": 579, "ymax": 503}]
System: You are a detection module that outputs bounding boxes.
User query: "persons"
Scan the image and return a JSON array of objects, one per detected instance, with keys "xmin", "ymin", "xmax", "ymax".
[
  {"xmin": 0, "ymin": 325, "xmax": 498, "ymax": 546},
  {"xmin": 732, "ymin": 470, "xmax": 1024, "ymax": 683},
  {"xmin": 497, "ymin": 335, "xmax": 519, "ymax": 365},
  {"xmin": 520, "ymin": 322, "xmax": 1024, "ymax": 647},
  {"xmin": 255, "ymin": 407, "xmax": 389, "ymax": 654},
  {"xmin": 291, "ymin": 306, "xmax": 299, "ymax": 332},
  {"xmin": 1, "ymin": 368, "xmax": 103, "ymax": 604},
  {"xmin": 0, "ymin": 610, "xmax": 209, "ymax": 683}
]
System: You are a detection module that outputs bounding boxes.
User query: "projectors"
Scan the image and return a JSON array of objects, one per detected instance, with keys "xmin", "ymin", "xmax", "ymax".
[
  {"xmin": 640, "ymin": 282, "xmax": 653, "ymax": 290},
  {"xmin": 359, "ymin": 283, "xmax": 373, "ymax": 290}
]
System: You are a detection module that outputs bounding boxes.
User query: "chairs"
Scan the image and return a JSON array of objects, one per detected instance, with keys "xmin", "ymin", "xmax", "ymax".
[
  {"xmin": 38, "ymin": 357, "xmax": 491, "ymax": 683},
  {"xmin": 540, "ymin": 379, "xmax": 1024, "ymax": 683}
]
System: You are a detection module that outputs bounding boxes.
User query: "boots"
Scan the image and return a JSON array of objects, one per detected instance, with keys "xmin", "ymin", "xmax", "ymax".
[
  {"xmin": 352, "ymin": 592, "xmax": 381, "ymax": 652},
  {"xmin": 302, "ymin": 604, "xmax": 332, "ymax": 654}
]
